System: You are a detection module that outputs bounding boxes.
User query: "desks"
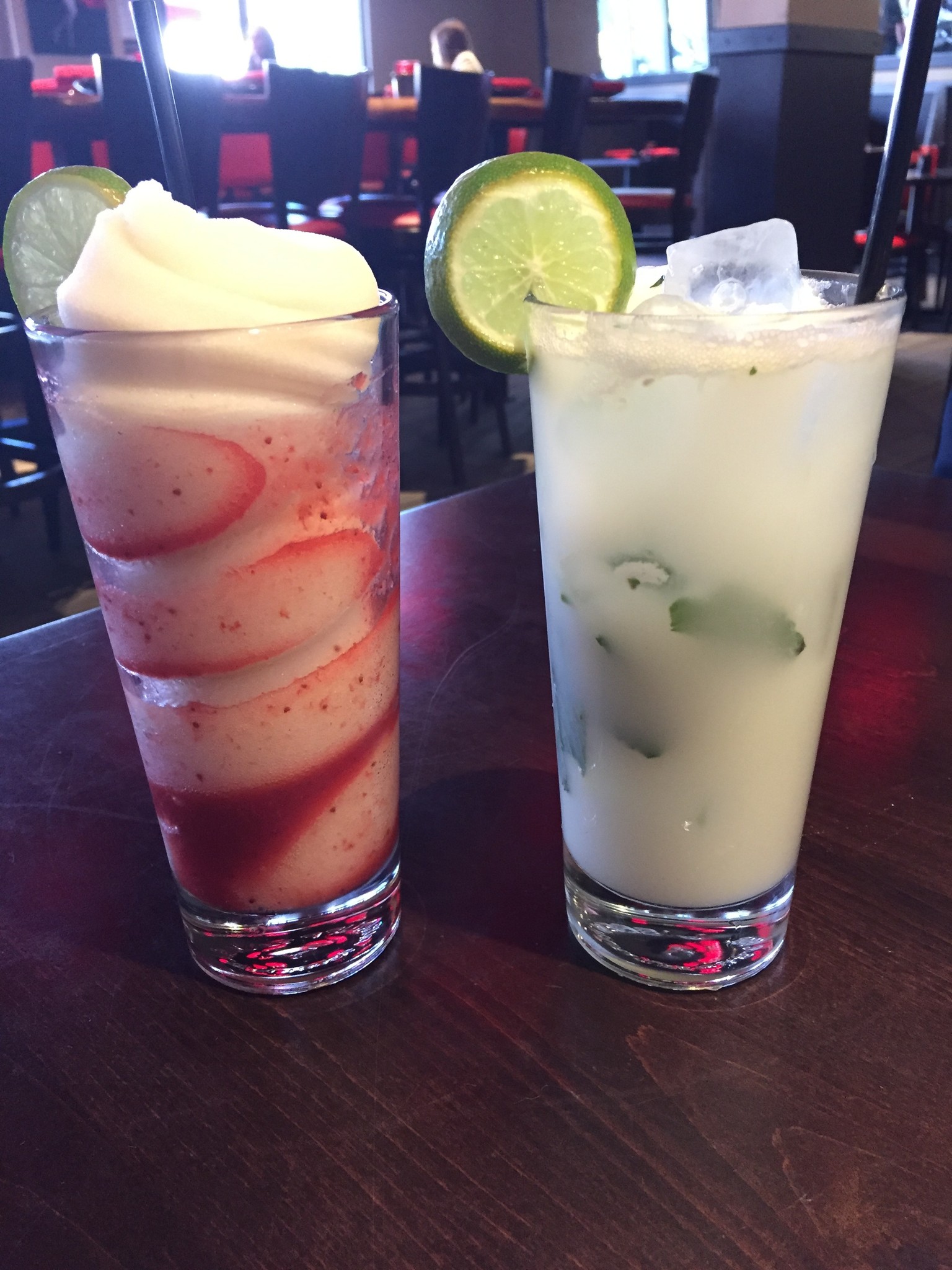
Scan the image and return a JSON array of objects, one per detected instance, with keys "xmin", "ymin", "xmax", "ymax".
[
  {"xmin": 35, "ymin": 95, "xmax": 685, "ymax": 191},
  {"xmin": 1, "ymin": 465, "xmax": 951, "ymax": 1269}
]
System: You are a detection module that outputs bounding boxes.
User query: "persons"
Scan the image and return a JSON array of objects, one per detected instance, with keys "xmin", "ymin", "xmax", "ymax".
[
  {"xmin": 429, "ymin": 18, "xmax": 485, "ymax": 74},
  {"xmin": 248, "ymin": 27, "xmax": 277, "ymax": 72}
]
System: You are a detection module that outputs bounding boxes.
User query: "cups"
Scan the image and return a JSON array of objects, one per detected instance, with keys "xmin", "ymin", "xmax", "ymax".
[
  {"xmin": 525, "ymin": 269, "xmax": 907, "ymax": 993},
  {"xmin": 23, "ymin": 290, "xmax": 402, "ymax": 996}
]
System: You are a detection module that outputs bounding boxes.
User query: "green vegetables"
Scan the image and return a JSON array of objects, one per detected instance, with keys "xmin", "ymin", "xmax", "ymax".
[{"xmin": 550, "ymin": 590, "xmax": 808, "ymax": 792}]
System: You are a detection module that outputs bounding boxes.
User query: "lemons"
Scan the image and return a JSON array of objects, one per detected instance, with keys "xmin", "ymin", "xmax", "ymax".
[
  {"xmin": 2, "ymin": 165, "xmax": 131, "ymax": 324},
  {"xmin": 425, "ymin": 151, "xmax": 637, "ymax": 375}
]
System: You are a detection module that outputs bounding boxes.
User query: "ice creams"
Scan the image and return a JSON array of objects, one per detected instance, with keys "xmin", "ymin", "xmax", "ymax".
[{"xmin": 38, "ymin": 179, "xmax": 398, "ymax": 919}]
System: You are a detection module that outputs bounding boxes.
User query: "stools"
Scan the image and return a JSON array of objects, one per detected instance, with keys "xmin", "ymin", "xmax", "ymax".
[{"xmin": 0, "ymin": 54, "xmax": 721, "ymax": 549}]
